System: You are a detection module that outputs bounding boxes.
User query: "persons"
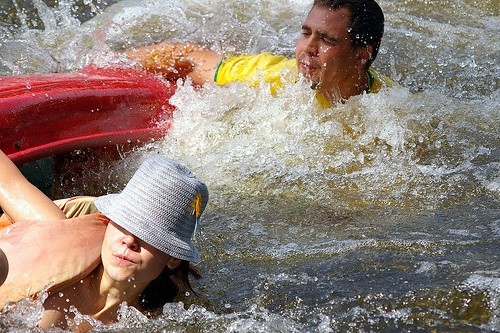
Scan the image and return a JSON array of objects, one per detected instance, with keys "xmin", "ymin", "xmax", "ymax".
[
  {"xmin": 101, "ymin": 0, "xmax": 402, "ymax": 117},
  {"xmin": 0, "ymin": 147, "xmax": 209, "ymax": 333}
]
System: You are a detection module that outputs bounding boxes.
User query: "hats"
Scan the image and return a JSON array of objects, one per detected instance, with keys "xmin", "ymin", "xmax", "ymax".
[{"xmin": 93, "ymin": 158, "xmax": 208, "ymax": 263}]
